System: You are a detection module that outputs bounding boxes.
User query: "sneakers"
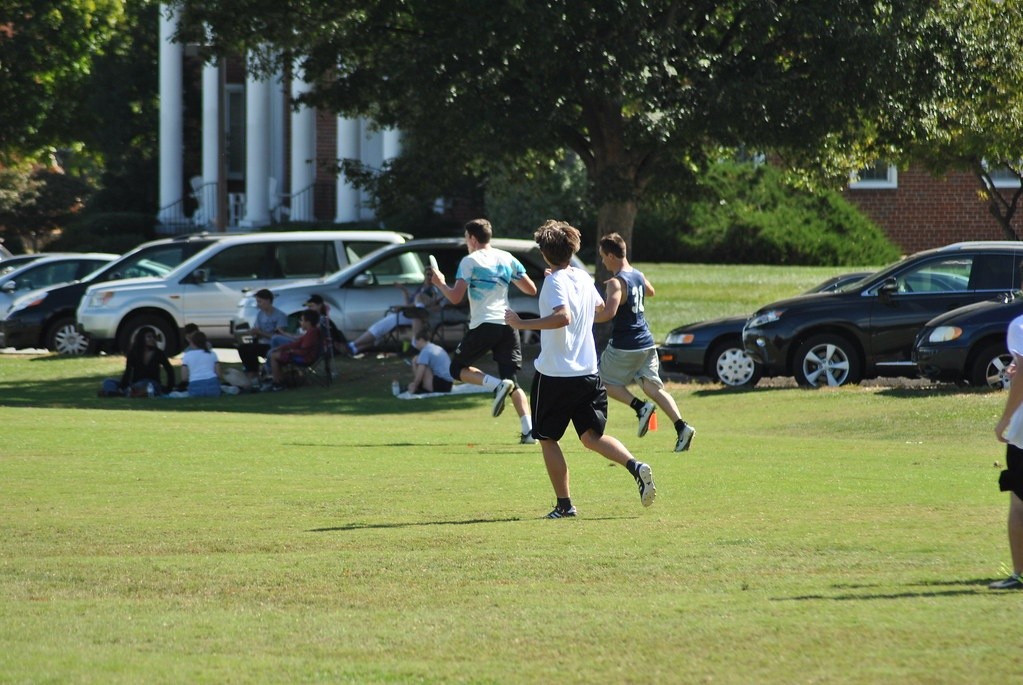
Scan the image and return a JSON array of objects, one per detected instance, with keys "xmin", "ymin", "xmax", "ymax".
[
  {"xmin": 673, "ymin": 424, "xmax": 696, "ymax": 451},
  {"xmin": 518, "ymin": 430, "xmax": 537, "ymax": 445},
  {"xmin": 630, "ymin": 463, "xmax": 655, "ymax": 507},
  {"xmin": 542, "ymin": 505, "xmax": 576, "ymax": 519},
  {"xmin": 492, "ymin": 379, "xmax": 514, "ymax": 418},
  {"xmin": 634, "ymin": 400, "xmax": 657, "ymax": 437}
]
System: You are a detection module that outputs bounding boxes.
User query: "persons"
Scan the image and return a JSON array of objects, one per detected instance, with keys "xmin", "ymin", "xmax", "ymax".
[
  {"xmin": 505, "ymin": 219, "xmax": 657, "ymax": 520},
  {"xmin": 117, "ymin": 290, "xmax": 333, "ymax": 398},
  {"xmin": 345, "ymin": 266, "xmax": 438, "ymax": 356},
  {"xmin": 429, "ymin": 219, "xmax": 539, "ymax": 445},
  {"xmin": 592, "ymin": 233, "xmax": 695, "ymax": 453},
  {"xmin": 988, "ymin": 315, "xmax": 1023, "ymax": 591},
  {"xmin": 407, "ymin": 327, "xmax": 453, "ymax": 396}
]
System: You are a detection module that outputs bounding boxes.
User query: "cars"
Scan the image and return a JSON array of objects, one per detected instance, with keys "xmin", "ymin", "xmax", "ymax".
[
  {"xmin": 912, "ymin": 287, "xmax": 1023, "ymax": 392},
  {"xmin": 658, "ymin": 268, "xmax": 972, "ymax": 390},
  {"xmin": 0, "ymin": 255, "xmax": 182, "ymax": 346}
]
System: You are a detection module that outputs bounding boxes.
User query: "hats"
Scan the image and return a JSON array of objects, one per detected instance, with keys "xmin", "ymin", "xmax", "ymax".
[{"xmin": 303, "ymin": 295, "xmax": 321, "ymax": 308}]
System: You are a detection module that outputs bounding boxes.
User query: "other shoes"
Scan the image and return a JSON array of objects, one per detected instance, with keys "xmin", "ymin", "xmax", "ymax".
[
  {"xmin": 987, "ymin": 574, "xmax": 1023, "ymax": 589},
  {"xmin": 218, "ymin": 383, "xmax": 239, "ymax": 396}
]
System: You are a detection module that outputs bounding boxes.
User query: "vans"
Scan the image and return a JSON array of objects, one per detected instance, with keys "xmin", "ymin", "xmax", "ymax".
[{"xmin": 0, "ymin": 233, "xmax": 248, "ymax": 359}]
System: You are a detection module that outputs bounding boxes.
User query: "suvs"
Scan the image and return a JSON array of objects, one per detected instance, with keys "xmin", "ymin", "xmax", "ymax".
[
  {"xmin": 230, "ymin": 237, "xmax": 589, "ymax": 378},
  {"xmin": 74, "ymin": 232, "xmax": 428, "ymax": 361},
  {"xmin": 741, "ymin": 239, "xmax": 1023, "ymax": 391}
]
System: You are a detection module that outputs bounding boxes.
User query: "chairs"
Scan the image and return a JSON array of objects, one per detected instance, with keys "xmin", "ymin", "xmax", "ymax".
[
  {"xmin": 285, "ymin": 323, "xmax": 333, "ymax": 391},
  {"xmin": 382, "ymin": 294, "xmax": 467, "ymax": 358}
]
